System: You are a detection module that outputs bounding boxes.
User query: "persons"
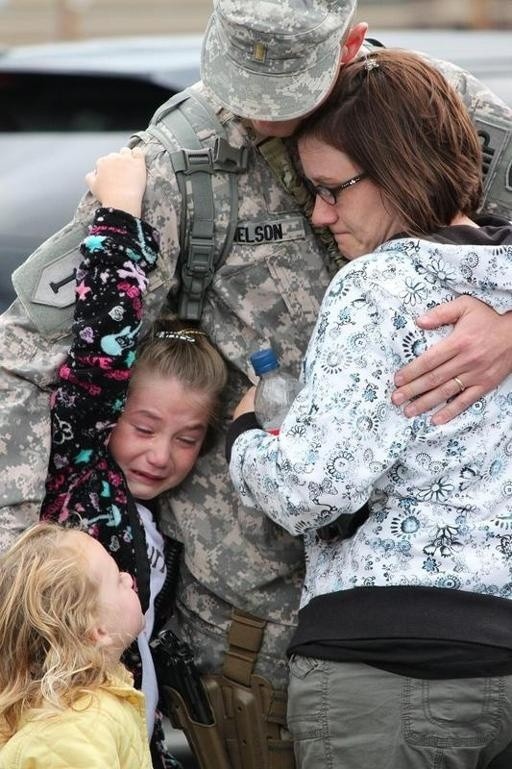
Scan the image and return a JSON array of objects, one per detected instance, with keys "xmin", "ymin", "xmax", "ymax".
[
  {"xmin": 1, "ymin": 521, "xmax": 158, "ymax": 768},
  {"xmin": 39, "ymin": 145, "xmax": 231, "ymax": 769},
  {"xmin": 0, "ymin": 0, "xmax": 511, "ymax": 768},
  {"xmin": 225, "ymin": 49, "xmax": 510, "ymax": 768}
]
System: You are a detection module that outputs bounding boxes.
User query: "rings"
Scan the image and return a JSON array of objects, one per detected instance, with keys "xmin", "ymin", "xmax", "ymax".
[{"xmin": 454, "ymin": 374, "xmax": 464, "ymax": 390}]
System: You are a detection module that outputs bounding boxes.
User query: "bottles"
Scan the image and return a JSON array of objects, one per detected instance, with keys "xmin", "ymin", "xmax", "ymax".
[{"xmin": 250, "ymin": 348, "xmax": 369, "ymax": 540}]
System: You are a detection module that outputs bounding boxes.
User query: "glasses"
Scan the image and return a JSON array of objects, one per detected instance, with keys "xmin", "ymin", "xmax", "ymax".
[{"xmin": 307, "ymin": 172, "xmax": 367, "ymax": 207}]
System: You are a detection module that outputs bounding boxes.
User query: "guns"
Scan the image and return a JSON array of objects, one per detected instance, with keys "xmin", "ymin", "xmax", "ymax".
[{"xmin": 150, "ymin": 628, "xmax": 215, "ymax": 725}]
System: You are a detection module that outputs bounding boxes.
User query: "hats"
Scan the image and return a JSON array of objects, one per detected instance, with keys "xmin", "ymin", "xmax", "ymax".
[{"xmin": 199, "ymin": 1, "xmax": 357, "ymax": 122}]
